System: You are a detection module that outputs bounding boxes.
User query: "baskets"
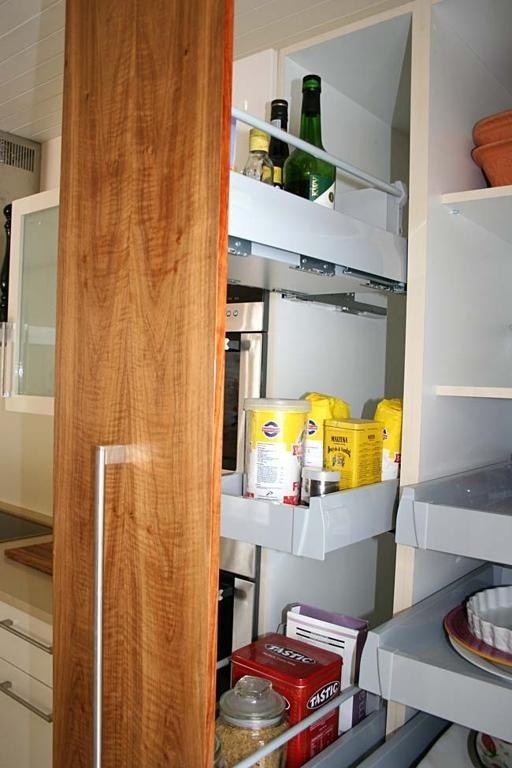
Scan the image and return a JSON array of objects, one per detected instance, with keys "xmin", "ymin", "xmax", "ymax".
[{"xmin": 471, "ymin": 110, "xmax": 512, "ymax": 187}]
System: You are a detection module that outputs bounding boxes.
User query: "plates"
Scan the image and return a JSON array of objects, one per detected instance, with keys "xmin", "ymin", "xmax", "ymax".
[
  {"xmin": 442, "ymin": 585, "xmax": 512, "ymax": 683},
  {"xmin": 466, "ymin": 729, "xmax": 512, "ymax": 768}
]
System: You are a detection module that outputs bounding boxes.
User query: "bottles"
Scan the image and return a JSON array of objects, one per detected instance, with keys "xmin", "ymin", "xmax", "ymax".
[
  {"xmin": 244, "ymin": 73, "xmax": 336, "ymax": 211},
  {"xmin": 213, "ymin": 675, "xmax": 289, "ymax": 767}
]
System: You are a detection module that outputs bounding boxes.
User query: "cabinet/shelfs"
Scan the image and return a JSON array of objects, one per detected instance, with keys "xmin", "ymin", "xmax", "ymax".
[
  {"xmin": 210, "ymin": 1, "xmax": 510, "ymax": 767},
  {"xmin": 5, "ymin": 189, "xmax": 60, "ymax": 417}
]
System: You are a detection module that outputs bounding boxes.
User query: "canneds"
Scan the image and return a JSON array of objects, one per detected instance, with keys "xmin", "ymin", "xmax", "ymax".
[{"xmin": 242, "ymin": 399, "xmax": 310, "ymax": 506}]
